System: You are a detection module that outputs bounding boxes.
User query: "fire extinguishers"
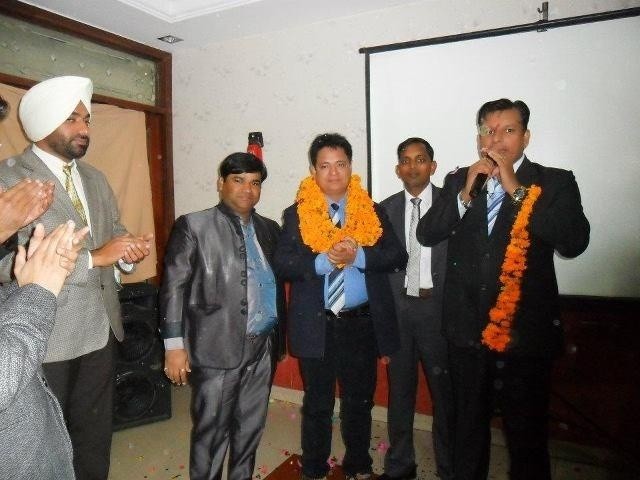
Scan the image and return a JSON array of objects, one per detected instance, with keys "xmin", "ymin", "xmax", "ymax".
[{"xmin": 247, "ymin": 132, "xmax": 264, "ymax": 162}]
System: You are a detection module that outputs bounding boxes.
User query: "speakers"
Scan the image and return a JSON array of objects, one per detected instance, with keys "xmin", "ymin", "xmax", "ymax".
[{"xmin": 113, "ymin": 281, "xmax": 171, "ymax": 431}]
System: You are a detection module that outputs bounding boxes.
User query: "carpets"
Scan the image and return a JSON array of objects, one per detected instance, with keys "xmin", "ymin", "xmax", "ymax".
[{"xmin": 263, "ymin": 455, "xmax": 382, "ymax": 480}]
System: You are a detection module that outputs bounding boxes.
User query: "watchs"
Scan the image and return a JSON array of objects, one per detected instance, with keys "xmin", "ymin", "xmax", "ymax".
[{"xmin": 511, "ymin": 185, "xmax": 526, "ymax": 207}]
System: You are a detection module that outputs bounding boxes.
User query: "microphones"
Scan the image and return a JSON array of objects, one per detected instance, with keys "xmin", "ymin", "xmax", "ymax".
[{"xmin": 468, "ymin": 153, "xmax": 497, "ymax": 197}]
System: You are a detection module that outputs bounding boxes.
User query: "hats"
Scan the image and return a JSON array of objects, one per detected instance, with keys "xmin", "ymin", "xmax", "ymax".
[{"xmin": 17, "ymin": 75, "xmax": 95, "ymax": 143}]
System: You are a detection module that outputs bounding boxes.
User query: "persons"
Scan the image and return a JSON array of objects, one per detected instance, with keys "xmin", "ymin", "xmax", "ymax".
[
  {"xmin": 0, "ymin": 219, "xmax": 90, "ymax": 479},
  {"xmin": 1, "ymin": 98, "xmax": 56, "ymax": 258},
  {"xmin": 158, "ymin": 152, "xmax": 286, "ymax": 480},
  {"xmin": 416, "ymin": 96, "xmax": 590, "ymax": 480},
  {"xmin": 272, "ymin": 133, "xmax": 408, "ymax": 479},
  {"xmin": 0, "ymin": 75, "xmax": 154, "ymax": 480},
  {"xmin": 378, "ymin": 138, "xmax": 453, "ymax": 479}
]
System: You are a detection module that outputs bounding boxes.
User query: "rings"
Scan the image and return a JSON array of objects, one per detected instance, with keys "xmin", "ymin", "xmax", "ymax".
[{"xmin": 180, "ymin": 367, "xmax": 186, "ymax": 372}]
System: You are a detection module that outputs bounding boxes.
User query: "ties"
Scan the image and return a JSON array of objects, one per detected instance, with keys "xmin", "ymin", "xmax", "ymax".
[
  {"xmin": 62, "ymin": 162, "xmax": 89, "ymax": 227},
  {"xmin": 487, "ymin": 179, "xmax": 506, "ymax": 237},
  {"xmin": 327, "ymin": 203, "xmax": 346, "ymax": 316},
  {"xmin": 406, "ymin": 198, "xmax": 423, "ymax": 297}
]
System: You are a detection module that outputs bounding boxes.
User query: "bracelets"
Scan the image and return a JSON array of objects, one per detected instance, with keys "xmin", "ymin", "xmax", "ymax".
[{"xmin": 459, "ymin": 192, "xmax": 474, "ymax": 210}]
